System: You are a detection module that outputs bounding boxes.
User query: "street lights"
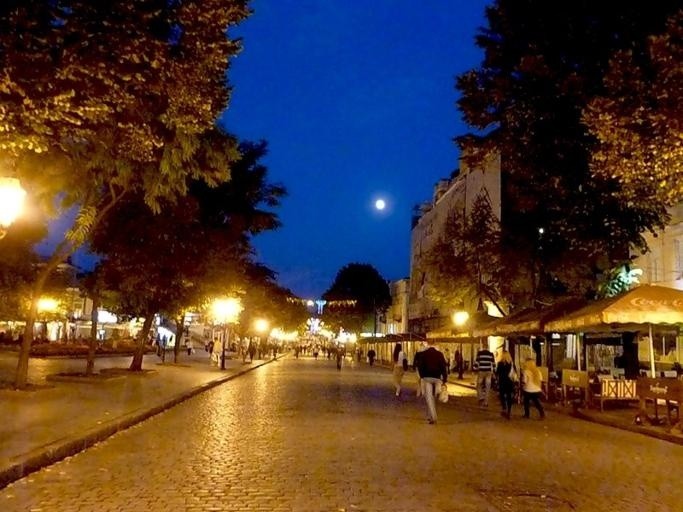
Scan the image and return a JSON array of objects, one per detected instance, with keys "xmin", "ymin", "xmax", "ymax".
[
  {"xmin": 454, "ymin": 313, "xmax": 468, "ymax": 379},
  {"xmin": 212, "ymin": 298, "xmax": 240, "ymax": 370},
  {"xmin": 252, "ymin": 320, "xmax": 300, "ymax": 363}
]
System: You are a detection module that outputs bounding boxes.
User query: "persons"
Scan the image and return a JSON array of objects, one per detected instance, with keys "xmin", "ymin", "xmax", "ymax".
[
  {"xmin": 186, "ymin": 337, "xmax": 463, "ymax": 396},
  {"xmin": 418, "ymin": 341, "xmax": 447, "ymax": 424},
  {"xmin": 520, "ymin": 359, "xmax": 545, "ymax": 418},
  {"xmin": 495, "ymin": 352, "xmax": 517, "ymax": 416},
  {"xmin": 473, "ymin": 344, "xmax": 495, "ymax": 406}
]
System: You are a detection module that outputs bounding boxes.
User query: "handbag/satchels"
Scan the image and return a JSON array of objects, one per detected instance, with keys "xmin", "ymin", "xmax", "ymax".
[
  {"xmin": 507, "ymin": 362, "xmax": 517, "ymax": 383},
  {"xmin": 402, "ymin": 353, "xmax": 408, "ymax": 371},
  {"xmin": 439, "ymin": 384, "xmax": 448, "ymax": 404}
]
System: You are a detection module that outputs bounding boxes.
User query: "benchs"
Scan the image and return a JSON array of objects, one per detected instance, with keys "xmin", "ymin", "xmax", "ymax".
[{"xmin": 594, "ymin": 378, "xmax": 636, "ymax": 413}]
